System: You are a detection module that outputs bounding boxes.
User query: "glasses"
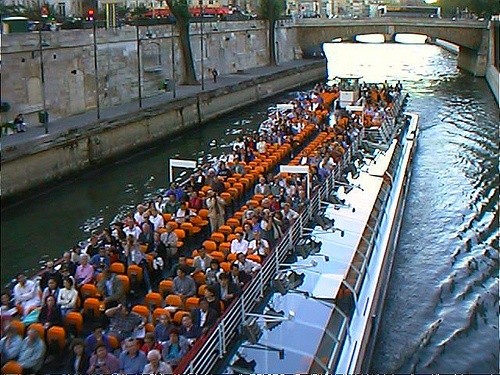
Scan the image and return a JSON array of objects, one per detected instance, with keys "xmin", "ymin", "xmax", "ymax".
[{"xmin": 60, "ymin": 270, "xmax": 68, "ymax": 273}]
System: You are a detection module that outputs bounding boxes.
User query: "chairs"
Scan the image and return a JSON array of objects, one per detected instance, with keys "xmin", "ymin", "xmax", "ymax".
[{"xmin": 0, "ymin": 87, "xmax": 396, "ymax": 374}]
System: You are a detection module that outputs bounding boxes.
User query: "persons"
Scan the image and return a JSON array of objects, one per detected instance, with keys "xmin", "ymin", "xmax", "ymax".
[
  {"xmin": 0, "ymin": 68, "xmax": 403, "ymax": 375},
  {"xmin": 470, "ymin": 12, "xmax": 478, "ymax": 20}
]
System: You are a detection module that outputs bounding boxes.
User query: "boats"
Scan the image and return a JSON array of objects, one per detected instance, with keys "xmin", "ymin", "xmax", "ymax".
[{"xmin": 0, "ymin": 80, "xmax": 422, "ymax": 375}]
